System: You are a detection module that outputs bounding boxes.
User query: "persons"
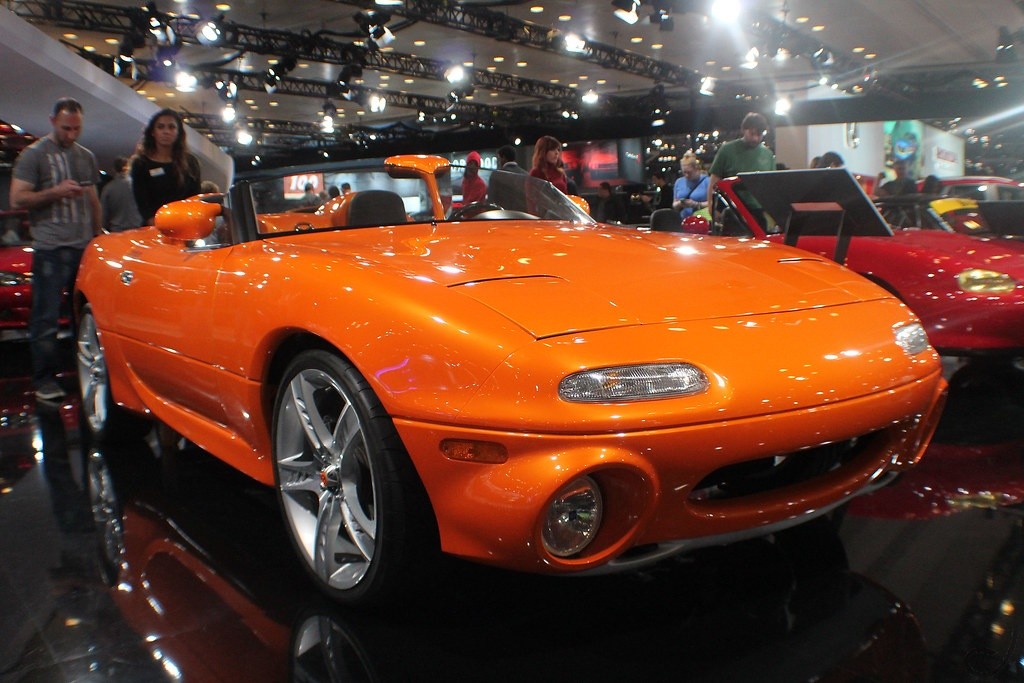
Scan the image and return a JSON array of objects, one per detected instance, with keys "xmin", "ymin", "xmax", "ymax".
[
  {"xmin": 303, "ymin": 113, "xmax": 940, "ymax": 237},
  {"xmin": 10, "ymin": 96, "xmax": 220, "ymax": 402},
  {"xmin": 30, "ymin": 403, "xmax": 103, "ymax": 606}
]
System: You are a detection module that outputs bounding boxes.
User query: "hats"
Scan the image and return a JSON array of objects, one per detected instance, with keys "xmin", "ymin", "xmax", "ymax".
[{"xmin": 466, "ymin": 150, "xmax": 480, "ymax": 167}]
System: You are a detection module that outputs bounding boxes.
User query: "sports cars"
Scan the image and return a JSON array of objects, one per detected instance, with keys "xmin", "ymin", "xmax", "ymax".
[
  {"xmin": 69, "ymin": 160, "xmax": 948, "ymax": 605},
  {"xmin": 649, "ymin": 167, "xmax": 1024, "ymax": 355}
]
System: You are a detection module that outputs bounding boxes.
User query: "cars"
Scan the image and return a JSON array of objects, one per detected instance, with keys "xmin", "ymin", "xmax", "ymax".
[{"xmin": 0, "ymin": 207, "xmax": 73, "ymax": 344}]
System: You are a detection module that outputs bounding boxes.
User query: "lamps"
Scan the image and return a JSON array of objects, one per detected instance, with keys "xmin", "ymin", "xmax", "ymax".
[{"xmin": 113, "ymin": 0, "xmax": 1024, "ymax": 166}]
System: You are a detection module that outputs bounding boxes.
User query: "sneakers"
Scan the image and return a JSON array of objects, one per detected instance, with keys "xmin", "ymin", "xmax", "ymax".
[{"xmin": 34, "ymin": 382, "xmax": 67, "ymax": 400}]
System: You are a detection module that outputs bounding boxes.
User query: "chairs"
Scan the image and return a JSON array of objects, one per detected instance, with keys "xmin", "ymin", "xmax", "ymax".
[
  {"xmin": 187, "ymin": 192, "xmax": 229, "ymax": 249},
  {"xmin": 337, "ymin": 190, "xmax": 409, "ymax": 228},
  {"xmin": 649, "ymin": 208, "xmax": 683, "ymax": 232},
  {"xmin": 721, "ymin": 206, "xmax": 768, "ymax": 236}
]
